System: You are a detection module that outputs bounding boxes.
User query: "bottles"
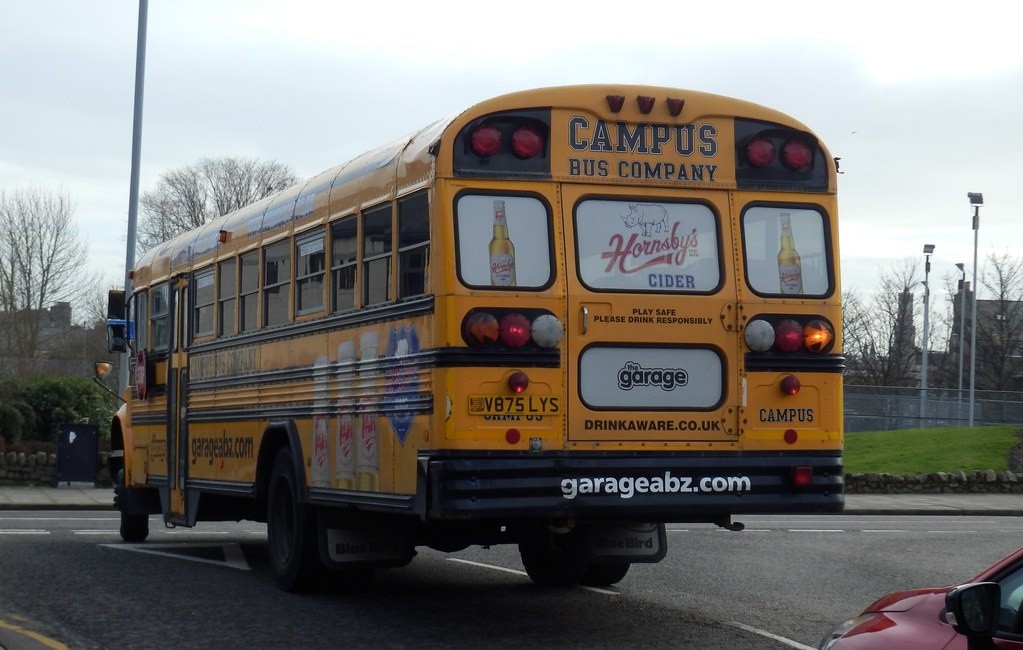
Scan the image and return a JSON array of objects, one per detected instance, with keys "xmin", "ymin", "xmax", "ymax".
[
  {"xmin": 778, "ymin": 214, "xmax": 803, "ymax": 294},
  {"xmin": 489, "ymin": 200, "xmax": 516, "ymax": 285}
]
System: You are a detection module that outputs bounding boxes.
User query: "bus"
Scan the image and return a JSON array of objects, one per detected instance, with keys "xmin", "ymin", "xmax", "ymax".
[{"xmin": 91, "ymin": 82, "xmax": 847, "ymax": 596}]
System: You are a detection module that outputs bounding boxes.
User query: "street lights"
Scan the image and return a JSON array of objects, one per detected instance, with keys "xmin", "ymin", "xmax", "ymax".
[
  {"xmin": 968, "ymin": 192, "xmax": 985, "ymax": 424},
  {"xmin": 918, "ymin": 244, "xmax": 935, "ymax": 426},
  {"xmin": 955, "ymin": 263, "xmax": 965, "ymax": 424}
]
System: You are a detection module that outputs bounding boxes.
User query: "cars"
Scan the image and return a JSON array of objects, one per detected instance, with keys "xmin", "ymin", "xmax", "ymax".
[{"xmin": 817, "ymin": 547, "xmax": 1023, "ymax": 650}]
